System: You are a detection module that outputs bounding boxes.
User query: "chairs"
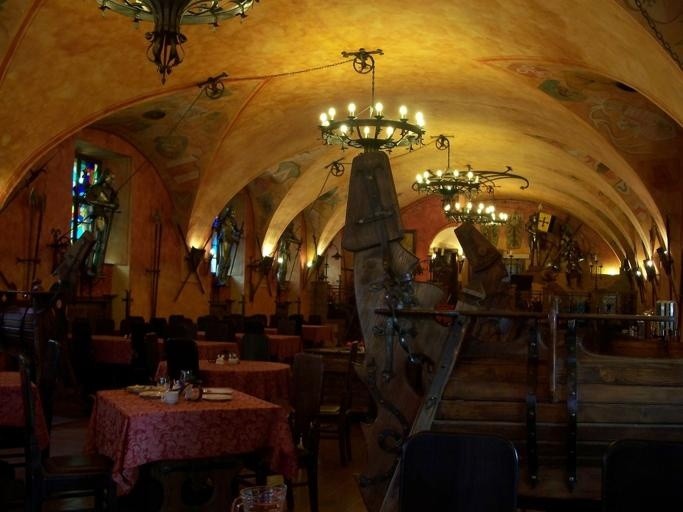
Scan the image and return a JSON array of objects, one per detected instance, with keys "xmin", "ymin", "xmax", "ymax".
[
  {"xmin": 600, "ymin": 439, "xmax": 683, "ymax": 512},
  {"xmin": 400, "ymin": 431, "xmax": 520, "ymax": 511}
]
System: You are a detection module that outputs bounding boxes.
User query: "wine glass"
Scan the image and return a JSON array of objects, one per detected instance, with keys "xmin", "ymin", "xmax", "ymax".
[{"xmin": 179, "ymin": 368, "xmax": 192, "ymax": 389}]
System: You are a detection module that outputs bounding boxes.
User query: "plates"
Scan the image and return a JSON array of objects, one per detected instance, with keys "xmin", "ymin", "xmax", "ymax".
[
  {"xmin": 141, "ymin": 391, "xmax": 164, "ymax": 398},
  {"xmin": 203, "ymin": 394, "xmax": 232, "ymax": 402},
  {"xmin": 205, "ymin": 388, "xmax": 234, "ymax": 395}
]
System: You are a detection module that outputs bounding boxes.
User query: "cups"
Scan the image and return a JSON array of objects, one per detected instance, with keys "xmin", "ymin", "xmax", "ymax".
[
  {"xmin": 229, "ymin": 359, "xmax": 238, "ymax": 364},
  {"xmin": 230, "ymin": 485, "xmax": 286, "ymax": 512},
  {"xmin": 162, "ymin": 392, "xmax": 180, "ymax": 405},
  {"xmin": 216, "ymin": 358, "xmax": 224, "ymax": 365}
]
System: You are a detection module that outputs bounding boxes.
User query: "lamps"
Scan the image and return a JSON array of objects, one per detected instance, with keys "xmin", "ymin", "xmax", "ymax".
[
  {"xmin": 411, "ymin": 134, "xmax": 509, "ymax": 228},
  {"xmin": 316, "ymin": 46, "xmax": 426, "ymax": 153},
  {"xmin": 89, "ymin": 1, "xmax": 265, "ymax": 82}
]
